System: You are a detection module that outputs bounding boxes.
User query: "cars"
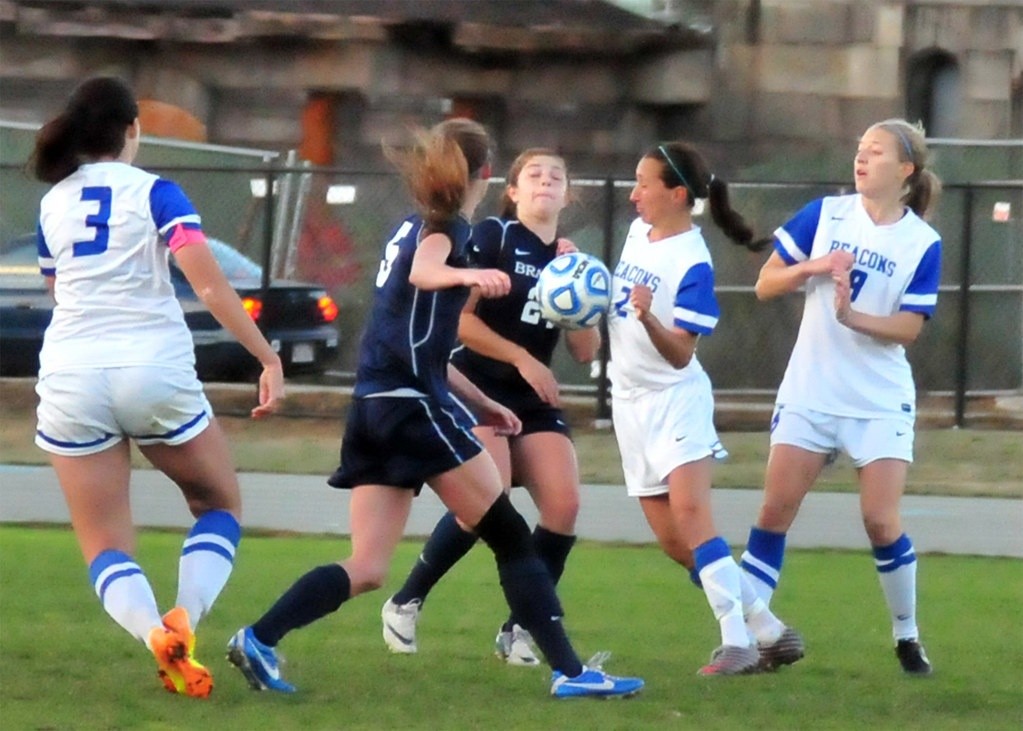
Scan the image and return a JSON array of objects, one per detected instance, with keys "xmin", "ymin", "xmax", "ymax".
[{"xmin": 0, "ymin": 231, "xmax": 339, "ymax": 384}]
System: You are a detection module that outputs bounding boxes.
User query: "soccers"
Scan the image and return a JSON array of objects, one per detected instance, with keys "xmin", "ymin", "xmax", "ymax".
[{"xmin": 534, "ymin": 251, "xmax": 614, "ymax": 331}]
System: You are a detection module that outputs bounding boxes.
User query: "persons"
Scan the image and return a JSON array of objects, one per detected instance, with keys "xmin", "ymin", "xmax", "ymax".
[
  {"xmin": 34, "ymin": 75, "xmax": 285, "ymax": 698},
  {"xmin": 225, "ymin": 115, "xmax": 802, "ymax": 698},
  {"xmin": 738, "ymin": 117, "xmax": 943, "ymax": 674}
]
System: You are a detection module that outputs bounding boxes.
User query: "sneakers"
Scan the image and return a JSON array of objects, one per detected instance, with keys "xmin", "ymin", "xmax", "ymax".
[
  {"xmin": 735, "ymin": 629, "xmax": 805, "ymax": 676},
  {"xmin": 149, "ymin": 606, "xmax": 214, "ymax": 700},
  {"xmin": 549, "ymin": 651, "xmax": 645, "ymax": 701},
  {"xmin": 695, "ymin": 642, "xmax": 766, "ymax": 677},
  {"xmin": 381, "ymin": 594, "xmax": 421, "ymax": 654},
  {"xmin": 224, "ymin": 626, "xmax": 298, "ymax": 694},
  {"xmin": 894, "ymin": 638, "xmax": 934, "ymax": 678},
  {"xmin": 495, "ymin": 623, "xmax": 541, "ymax": 667}
]
